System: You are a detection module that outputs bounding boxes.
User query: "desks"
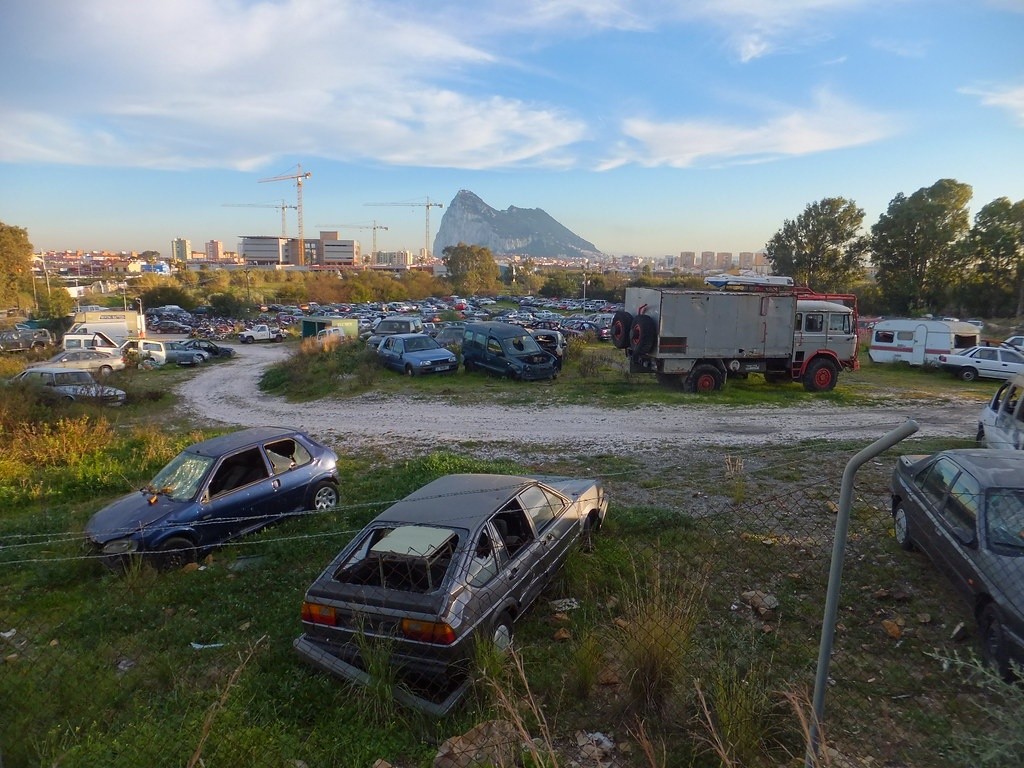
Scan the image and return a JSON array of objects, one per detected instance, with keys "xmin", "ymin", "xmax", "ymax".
[{"xmin": 370, "ymin": 525, "xmax": 455, "ymax": 588}]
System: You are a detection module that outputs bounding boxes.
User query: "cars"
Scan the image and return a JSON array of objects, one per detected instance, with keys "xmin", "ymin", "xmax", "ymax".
[
  {"xmin": 153, "ymin": 321, "xmax": 192, "ymax": 334},
  {"xmin": 518, "ymin": 310, "xmax": 616, "ymax": 340},
  {"xmin": 968, "ymin": 320, "xmax": 984, "ymax": 330},
  {"xmin": 1000, "ymin": 336, "xmax": 1024, "ymax": 354},
  {"xmin": 892, "ymin": 449, "xmax": 1024, "ymax": 685},
  {"xmin": 163, "ymin": 337, "xmax": 236, "ymax": 365},
  {"xmin": 27, "ymin": 349, "xmax": 127, "ymax": 377},
  {"xmin": 256, "ymin": 293, "xmax": 517, "ymax": 326},
  {"xmin": 378, "ymin": 334, "xmax": 460, "ymax": 378},
  {"xmin": 82, "ymin": 426, "xmax": 340, "ymax": 571},
  {"xmin": 938, "ymin": 345, "xmax": 1024, "ymax": 380},
  {"xmin": 3, "ymin": 367, "xmax": 126, "ymax": 409},
  {"xmin": 520, "ymin": 295, "xmax": 624, "ymax": 313},
  {"xmin": 293, "ymin": 473, "xmax": 611, "ymax": 717}
]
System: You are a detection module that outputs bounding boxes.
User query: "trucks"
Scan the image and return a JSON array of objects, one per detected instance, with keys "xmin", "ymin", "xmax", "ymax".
[
  {"xmin": 610, "ymin": 277, "xmax": 862, "ymax": 394},
  {"xmin": 62, "ymin": 312, "xmax": 139, "ymax": 345}
]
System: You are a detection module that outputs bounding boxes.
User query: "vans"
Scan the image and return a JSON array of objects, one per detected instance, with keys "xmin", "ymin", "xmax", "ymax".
[
  {"xmin": 1, "ymin": 328, "xmax": 55, "ymax": 352},
  {"xmin": 463, "ymin": 322, "xmax": 559, "ymax": 383},
  {"xmin": 62, "ymin": 335, "xmax": 98, "ymax": 348}
]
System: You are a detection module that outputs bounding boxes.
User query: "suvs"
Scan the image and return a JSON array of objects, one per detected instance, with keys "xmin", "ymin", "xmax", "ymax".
[
  {"xmin": 89, "ymin": 331, "xmax": 166, "ymax": 369},
  {"xmin": 366, "ymin": 317, "xmax": 424, "ymax": 356}
]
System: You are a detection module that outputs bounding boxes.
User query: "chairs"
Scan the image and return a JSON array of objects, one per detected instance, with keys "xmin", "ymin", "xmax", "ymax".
[{"xmin": 492, "ymin": 519, "xmax": 524, "ymax": 555}]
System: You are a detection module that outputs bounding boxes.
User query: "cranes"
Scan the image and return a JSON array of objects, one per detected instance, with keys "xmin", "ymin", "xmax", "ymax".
[
  {"xmin": 317, "ymin": 220, "xmax": 388, "ymax": 267},
  {"xmin": 256, "ymin": 162, "xmax": 311, "ymax": 266},
  {"xmin": 222, "ymin": 199, "xmax": 298, "ymax": 238},
  {"xmin": 363, "ymin": 196, "xmax": 443, "ymax": 261}
]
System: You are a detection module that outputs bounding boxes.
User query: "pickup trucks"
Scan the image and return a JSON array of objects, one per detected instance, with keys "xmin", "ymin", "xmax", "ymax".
[{"xmin": 241, "ymin": 325, "xmax": 287, "ymax": 342}]
